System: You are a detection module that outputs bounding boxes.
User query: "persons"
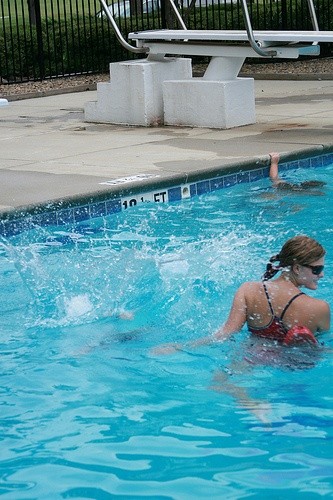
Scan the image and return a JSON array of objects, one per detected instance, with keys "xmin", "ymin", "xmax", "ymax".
[
  {"xmin": 261, "ymin": 151, "xmax": 324, "ymax": 212},
  {"xmin": 150, "ymin": 233, "xmax": 330, "ymax": 426}
]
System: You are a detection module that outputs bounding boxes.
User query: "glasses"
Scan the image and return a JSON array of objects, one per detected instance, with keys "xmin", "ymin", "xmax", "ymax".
[{"xmin": 298, "ymin": 263, "xmax": 325, "ymax": 275}]
